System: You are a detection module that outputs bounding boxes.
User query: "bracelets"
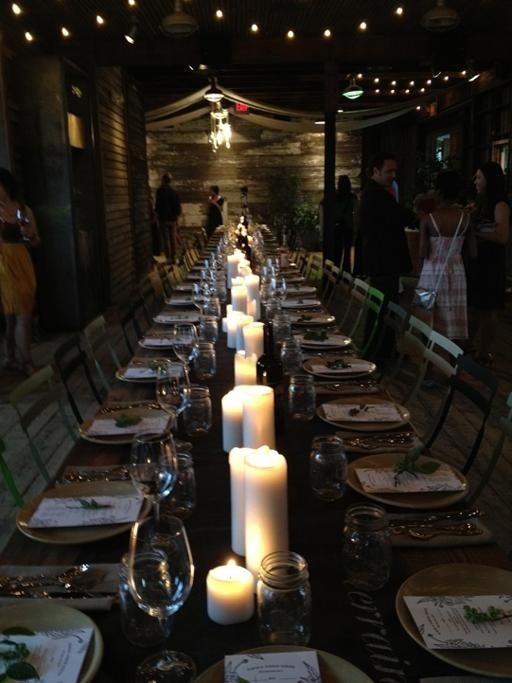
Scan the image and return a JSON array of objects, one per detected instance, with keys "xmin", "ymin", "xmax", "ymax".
[{"xmin": 29, "ymin": 232, "xmax": 37, "ymax": 240}]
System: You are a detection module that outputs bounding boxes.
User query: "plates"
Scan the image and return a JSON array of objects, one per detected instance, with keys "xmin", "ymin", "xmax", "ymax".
[
  {"xmin": 0, "ymin": 231, "xmax": 225, "ymax": 683},
  {"xmin": 191, "ymin": 231, "xmax": 511, "ymax": 683}
]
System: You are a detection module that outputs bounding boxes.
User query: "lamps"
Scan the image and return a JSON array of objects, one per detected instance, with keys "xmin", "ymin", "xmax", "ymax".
[
  {"xmin": 202, "ymin": 70, "xmax": 235, "ymax": 153},
  {"xmin": 342, "ymin": 76, "xmax": 364, "ymax": 102},
  {"xmin": 124, "ymin": 24, "xmax": 141, "ymax": 45}
]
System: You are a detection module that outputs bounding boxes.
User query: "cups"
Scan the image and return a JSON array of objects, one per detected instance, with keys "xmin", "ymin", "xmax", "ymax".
[{"xmin": 158, "ymin": 284, "xmax": 394, "ymax": 645}]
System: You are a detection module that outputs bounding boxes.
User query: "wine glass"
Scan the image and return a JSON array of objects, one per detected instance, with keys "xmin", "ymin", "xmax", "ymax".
[
  {"xmin": 16, "ymin": 207, "xmax": 33, "ymax": 242},
  {"xmin": 128, "ymin": 231, "xmax": 236, "ymax": 683}
]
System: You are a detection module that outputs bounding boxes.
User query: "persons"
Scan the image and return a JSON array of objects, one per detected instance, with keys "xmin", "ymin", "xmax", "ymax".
[
  {"xmin": 319, "ymin": 175, "xmax": 357, "ymax": 276},
  {"xmin": 0, "ymin": 166, "xmax": 42, "ymax": 377},
  {"xmin": 358, "ymin": 152, "xmax": 510, "ymax": 369},
  {"xmin": 207, "ymin": 185, "xmax": 224, "ymax": 242},
  {"xmin": 155, "ymin": 173, "xmax": 182, "ymax": 265}
]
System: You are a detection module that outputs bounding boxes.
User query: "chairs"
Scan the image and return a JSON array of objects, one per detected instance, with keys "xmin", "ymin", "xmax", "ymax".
[
  {"xmin": 2, "ymin": 239, "xmax": 203, "ymax": 552},
  {"xmin": 292, "ymin": 247, "xmax": 509, "ymax": 544}
]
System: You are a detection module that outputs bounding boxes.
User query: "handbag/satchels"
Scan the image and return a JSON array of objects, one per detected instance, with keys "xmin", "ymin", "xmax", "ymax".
[{"xmin": 411, "ymin": 288, "xmax": 436, "ymax": 315}]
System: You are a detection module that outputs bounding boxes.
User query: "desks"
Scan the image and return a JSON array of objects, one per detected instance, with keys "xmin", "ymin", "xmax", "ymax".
[{"xmin": 2, "ymin": 222, "xmax": 509, "ymax": 682}]
